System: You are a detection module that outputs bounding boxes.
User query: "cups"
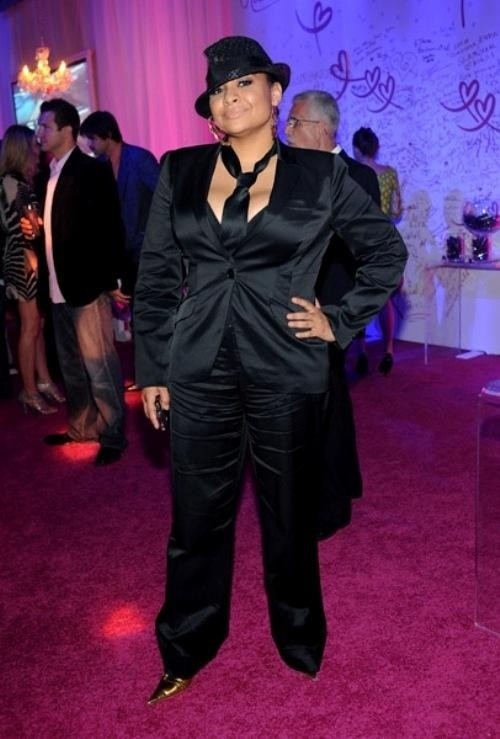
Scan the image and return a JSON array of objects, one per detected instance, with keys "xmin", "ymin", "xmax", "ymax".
[
  {"xmin": 23, "ymin": 203, "xmax": 41, "ymax": 237},
  {"xmin": 444, "ymin": 232, "xmax": 465, "ymax": 263}
]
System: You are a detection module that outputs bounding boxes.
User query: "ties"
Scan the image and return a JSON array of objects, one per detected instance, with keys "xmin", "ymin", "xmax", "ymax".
[{"xmin": 221, "ymin": 133, "xmax": 278, "ymax": 251}]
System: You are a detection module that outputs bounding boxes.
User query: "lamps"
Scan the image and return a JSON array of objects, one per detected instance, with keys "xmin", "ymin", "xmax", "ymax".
[{"xmin": 15, "ymin": 31, "xmax": 70, "ymax": 102}]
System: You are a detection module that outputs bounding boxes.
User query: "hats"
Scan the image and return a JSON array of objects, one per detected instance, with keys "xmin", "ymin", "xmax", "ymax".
[{"xmin": 194, "ymin": 36, "xmax": 291, "ymax": 120}]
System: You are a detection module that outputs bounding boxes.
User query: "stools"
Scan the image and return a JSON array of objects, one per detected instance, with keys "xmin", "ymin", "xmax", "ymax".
[{"xmin": 471, "ymin": 377, "xmax": 500, "ymax": 637}]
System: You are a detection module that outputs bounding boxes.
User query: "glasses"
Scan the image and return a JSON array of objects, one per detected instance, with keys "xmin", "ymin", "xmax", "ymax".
[{"xmin": 287, "ymin": 118, "xmax": 320, "ymax": 127}]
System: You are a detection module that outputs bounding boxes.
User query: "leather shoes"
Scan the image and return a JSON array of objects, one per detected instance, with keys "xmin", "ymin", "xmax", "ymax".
[
  {"xmin": 94, "ymin": 439, "xmax": 129, "ymax": 467},
  {"xmin": 42, "ymin": 432, "xmax": 73, "ymax": 445},
  {"xmin": 147, "ymin": 671, "xmax": 194, "ymax": 704}
]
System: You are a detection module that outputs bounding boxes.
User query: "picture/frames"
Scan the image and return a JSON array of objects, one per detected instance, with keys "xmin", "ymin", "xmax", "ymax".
[{"xmin": 7, "ymin": 47, "xmax": 99, "ymax": 148}]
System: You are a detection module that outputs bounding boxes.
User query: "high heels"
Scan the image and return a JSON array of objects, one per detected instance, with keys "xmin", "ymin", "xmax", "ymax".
[
  {"xmin": 37, "ymin": 383, "xmax": 66, "ymax": 404},
  {"xmin": 18, "ymin": 390, "xmax": 59, "ymax": 415},
  {"xmin": 378, "ymin": 353, "xmax": 393, "ymax": 375},
  {"xmin": 355, "ymin": 353, "xmax": 367, "ymax": 379}
]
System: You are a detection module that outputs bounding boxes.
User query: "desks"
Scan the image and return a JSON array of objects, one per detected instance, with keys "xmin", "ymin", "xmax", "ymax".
[{"xmin": 421, "ymin": 257, "xmax": 500, "ymax": 371}]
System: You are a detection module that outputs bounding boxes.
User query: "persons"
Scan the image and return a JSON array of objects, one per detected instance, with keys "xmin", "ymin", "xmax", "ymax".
[
  {"xmin": 0, "ymin": 103, "xmax": 159, "ymax": 469},
  {"xmin": 353, "ymin": 126, "xmax": 403, "ymax": 376},
  {"xmin": 130, "ymin": 37, "xmax": 410, "ymax": 705},
  {"xmin": 283, "ymin": 91, "xmax": 380, "ymax": 540}
]
